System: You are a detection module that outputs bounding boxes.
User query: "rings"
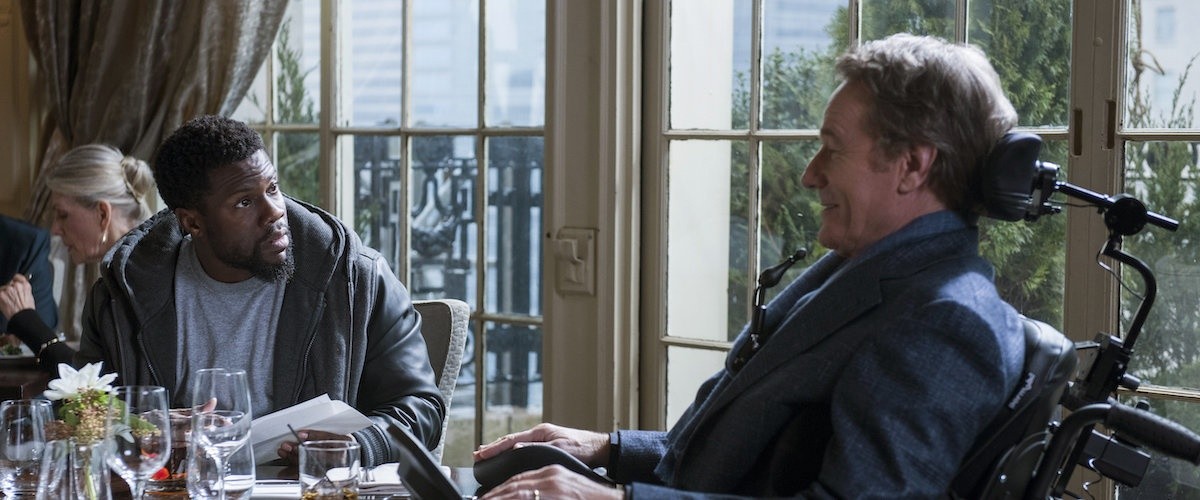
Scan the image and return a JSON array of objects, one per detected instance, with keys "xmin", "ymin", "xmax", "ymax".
[{"xmin": 534, "ymin": 489, "xmax": 540, "ymax": 500}]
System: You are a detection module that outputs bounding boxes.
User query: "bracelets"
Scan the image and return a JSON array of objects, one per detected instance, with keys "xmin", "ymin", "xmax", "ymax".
[{"xmin": 37, "ymin": 336, "xmax": 65, "ymax": 364}]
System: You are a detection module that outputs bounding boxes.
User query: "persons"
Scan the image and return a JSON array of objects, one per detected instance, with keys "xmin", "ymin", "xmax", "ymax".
[
  {"xmin": 77, "ymin": 116, "xmax": 447, "ymax": 472},
  {"xmin": 472, "ymin": 29, "xmax": 1026, "ymax": 500},
  {"xmin": 0, "ymin": 143, "xmax": 154, "ymax": 407}
]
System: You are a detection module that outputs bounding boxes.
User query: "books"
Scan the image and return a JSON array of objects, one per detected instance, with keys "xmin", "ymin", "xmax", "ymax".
[{"xmin": 252, "ymin": 463, "xmax": 408, "ymax": 500}]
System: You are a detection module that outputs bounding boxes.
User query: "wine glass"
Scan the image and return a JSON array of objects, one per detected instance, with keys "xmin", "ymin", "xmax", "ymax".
[
  {"xmin": 191, "ymin": 367, "xmax": 252, "ymax": 500},
  {"xmin": 104, "ymin": 386, "xmax": 171, "ymax": 500}
]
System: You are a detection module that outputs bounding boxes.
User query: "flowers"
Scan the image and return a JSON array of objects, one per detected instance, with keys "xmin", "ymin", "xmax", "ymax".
[{"xmin": 42, "ymin": 361, "xmax": 146, "ymax": 442}]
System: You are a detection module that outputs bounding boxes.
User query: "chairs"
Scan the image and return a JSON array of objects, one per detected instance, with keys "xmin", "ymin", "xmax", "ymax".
[{"xmin": 409, "ymin": 299, "xmax": 471, "ymax": 461}]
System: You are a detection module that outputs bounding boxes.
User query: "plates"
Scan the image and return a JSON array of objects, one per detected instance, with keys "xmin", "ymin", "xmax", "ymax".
[{"xmin": 0, "ymin": 342, "xmax": 36, "ymax": 359}]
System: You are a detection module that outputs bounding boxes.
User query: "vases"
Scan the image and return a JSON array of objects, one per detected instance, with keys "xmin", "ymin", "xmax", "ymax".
[{"xmin": 66, "ymin": 440, "xmax": 113, "ymax": 500}]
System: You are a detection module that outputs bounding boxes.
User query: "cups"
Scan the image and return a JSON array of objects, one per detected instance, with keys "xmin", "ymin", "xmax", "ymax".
[
  {"xmin": 34, "ymin": 437, "xmax": 113, "ymax": 500},
  {"xmin": 186, "ymin": 412, "xmax": 256, "ymax": 500},
  {"xmin": 298, "ymin": 440, "xmax": 361, "ymax": 500},
  {"xmin": 0, "ymin": 399, "xmax": 61, "ymax": 497}
]
